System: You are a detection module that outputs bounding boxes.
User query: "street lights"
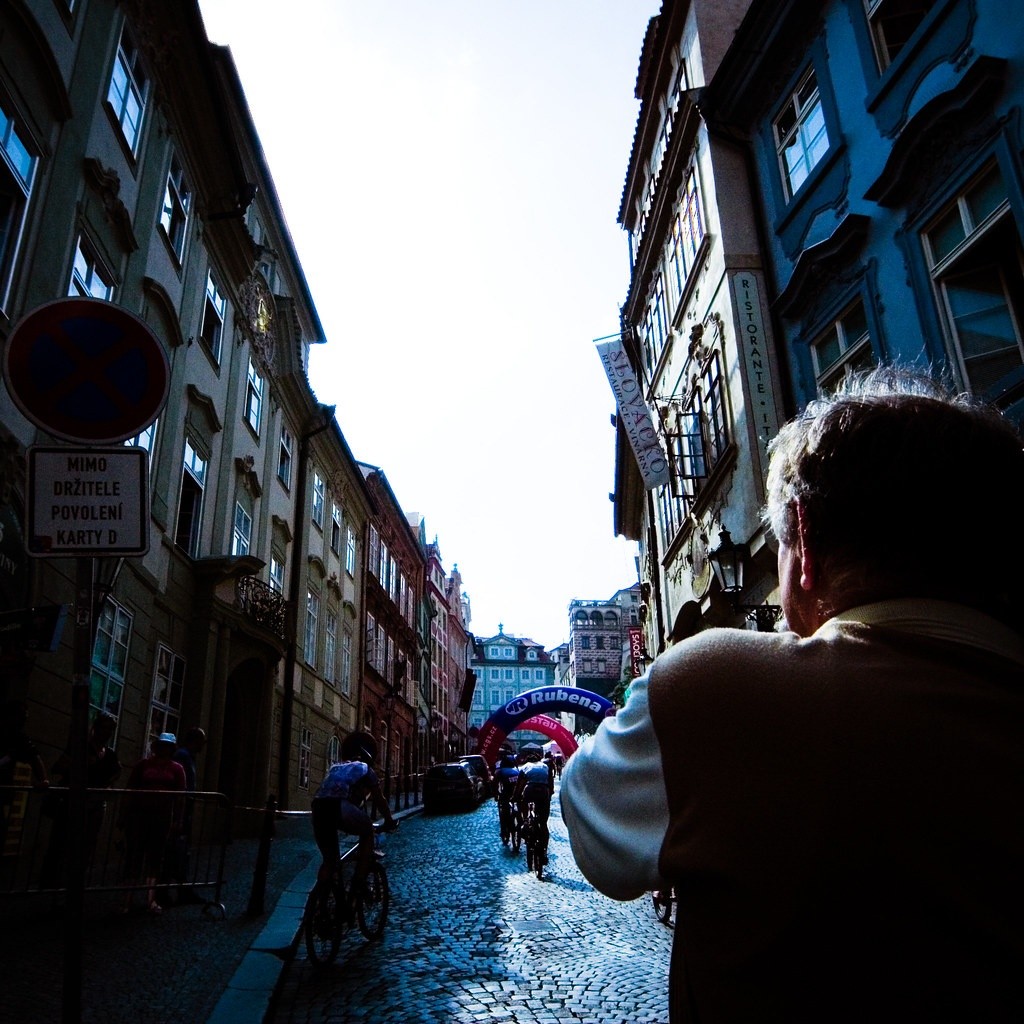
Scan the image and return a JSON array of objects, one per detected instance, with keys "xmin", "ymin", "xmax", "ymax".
[
  {"xmin": 432, "ymin": 713, "xmax": 442, "ymax": 764},
  {"xmin": 383, "ymin": 689, "xmax": 398, "ymax": 815}
]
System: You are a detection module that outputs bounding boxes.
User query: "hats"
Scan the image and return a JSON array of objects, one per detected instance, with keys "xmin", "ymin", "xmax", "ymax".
[{"xmin": 151, "ymin": 732, "xmax": 177, "ymax": 753}]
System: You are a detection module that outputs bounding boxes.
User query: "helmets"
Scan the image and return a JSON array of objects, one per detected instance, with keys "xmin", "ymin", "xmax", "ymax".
[{"xmin": 501, "ymin": 755, "xmax": 515, "ymax": 767}]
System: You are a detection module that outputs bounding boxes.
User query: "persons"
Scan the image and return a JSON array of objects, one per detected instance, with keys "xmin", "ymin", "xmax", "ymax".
[
  {"xmin": 560, "ymin": 384, "xmax": 1024, "ymax": 1024},
  {"xmin": 114, "ymin": 730, "xmax": 207, "ymax": 914},
  {"xmin": 41, "ymin": 715, "xmax": 123, "ymax": 929},
  {"xmin": 492, "ymin": 749, "xmax": 564, "ymax": 865},
  {"xmin": 312, "ymin": 731, "xmax": 396, "ymax": 938}
]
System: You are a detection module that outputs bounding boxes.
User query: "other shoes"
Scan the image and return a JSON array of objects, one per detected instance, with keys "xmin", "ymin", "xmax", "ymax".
[
  {"xmin": 118, "ymin": 904, "xmax": 128, "ymax": 914},
  {"xmin": 146, "ymin": 899, "xmax": 163, "ymax": 913}
]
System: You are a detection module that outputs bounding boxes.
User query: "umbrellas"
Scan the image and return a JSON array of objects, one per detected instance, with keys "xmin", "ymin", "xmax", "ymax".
[{"xmin": 521, "ymin": 742, "xmax": 540, "ymax": 749}]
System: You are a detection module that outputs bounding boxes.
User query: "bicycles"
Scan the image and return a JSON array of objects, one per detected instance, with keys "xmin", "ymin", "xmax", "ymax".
[
  {"xmin": 305, "ymin": 821, "xmax": 400, "ymax": 973},
  {"xmin": 650, "ymin": 889, "xmax": 678, "ymax": 924},
  {"xmin": 492, "ymin": 791, "xmax": 522, "ymax": 853},
  {"xmin": 519, "ymin": 800, "xmax": 544, "ymax": 879}
]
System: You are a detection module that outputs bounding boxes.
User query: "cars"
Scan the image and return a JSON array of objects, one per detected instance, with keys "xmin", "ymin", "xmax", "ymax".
[{"xmin": 421, "ymin": 762, "xmax": 485, "ymax": 810}]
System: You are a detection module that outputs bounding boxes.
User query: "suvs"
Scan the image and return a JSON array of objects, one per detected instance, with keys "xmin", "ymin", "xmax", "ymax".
[{"xmin": 452, "ymin": 754, "xmax": 494, "ymax": 798}]
